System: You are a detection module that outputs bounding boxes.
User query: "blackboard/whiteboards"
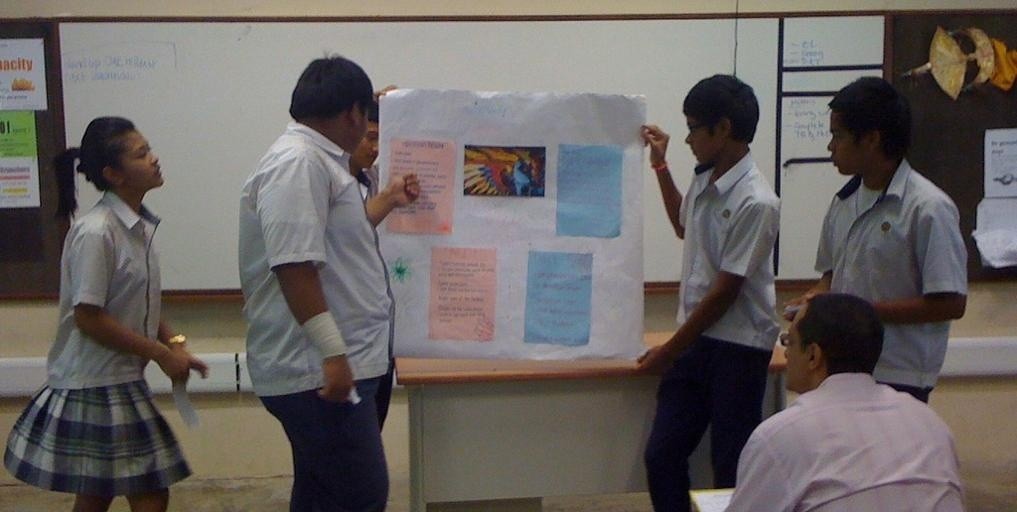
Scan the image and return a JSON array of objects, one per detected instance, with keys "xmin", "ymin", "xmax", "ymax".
[{"xmin": 50, "ymin": 14, "xmax": 894, "ymax": 304}]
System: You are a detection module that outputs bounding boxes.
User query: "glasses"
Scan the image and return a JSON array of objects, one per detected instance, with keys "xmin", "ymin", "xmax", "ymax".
[
  {"xmin": 363, "ymin": 135, "xmax": 378, "ymax": 145},
  {"xmin": 689, "ymin": 122, "xmax": 703, "ymax": 134},
  {"xmin": 780, "ymin": 333, "xmax": 804, "ymax": 346}
]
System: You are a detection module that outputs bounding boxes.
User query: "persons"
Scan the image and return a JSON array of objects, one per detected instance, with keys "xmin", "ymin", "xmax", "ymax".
[
  {"xmin": 43, "ymin": 116, "xmax": 210, "ymax": 511},
  {"xmin": 781, "ymin": 75, "xmax": 969, "ymax": 404},
  {"xmin": 724, "ymin": 291, "xmax": 968, "ymax": 511},
  {"xmin": 635, "ymin": 74, "xmax": 782, "ymax": 512},
  {"xmin": 239, "ymin": 58, "xmax": 420, "ymax": 510},
  {"xmin": 348, "ymin": 85, "xmax": 399, "ymax": 429}
]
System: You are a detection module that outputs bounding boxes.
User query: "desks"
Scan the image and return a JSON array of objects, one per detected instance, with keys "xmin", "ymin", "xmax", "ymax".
[{"xmin": 394, "ymin": 333, "xmax": 788, "ymax": 512}]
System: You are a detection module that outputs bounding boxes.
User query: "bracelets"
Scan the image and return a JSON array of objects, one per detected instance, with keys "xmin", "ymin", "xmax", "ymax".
[
  {"xmin": 651, "ymin": 163, "xmax": 668, "ymax": 171},
  {"xmin": 167, "ymin": 334, "xmax": 188, "ymax": 345}
]
